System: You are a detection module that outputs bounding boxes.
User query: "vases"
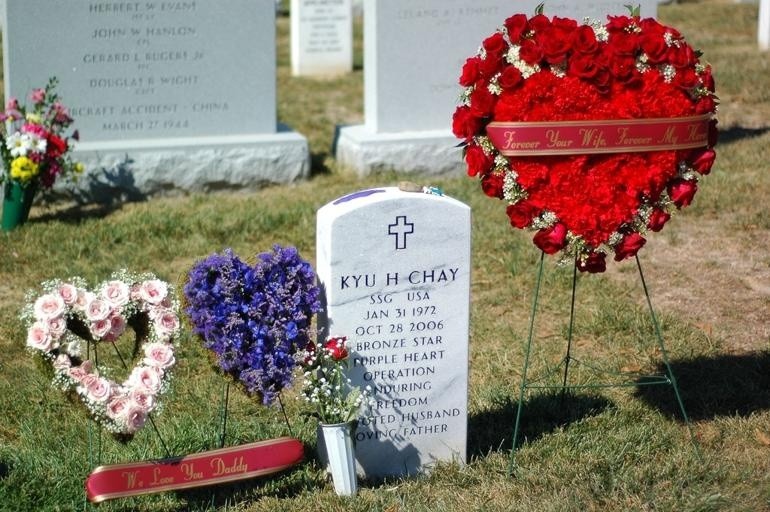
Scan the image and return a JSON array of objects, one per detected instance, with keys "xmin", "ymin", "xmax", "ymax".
[
  {"xmin": 2, "ymin": 181, "xmax": 36, "ymax": 233},
  {"xmin": 317, "ymin": 420, "xmax": 359, "ymax": 497}
]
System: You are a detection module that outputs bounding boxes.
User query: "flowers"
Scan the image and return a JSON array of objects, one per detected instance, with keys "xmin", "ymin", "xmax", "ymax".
[
  {"xmin": 452, "ymin": 3, "xmax": 717, "ymax": 276},
  {"xmin": 0, "ymin": 75, "xmax": 83, "ymax": 206}
]
